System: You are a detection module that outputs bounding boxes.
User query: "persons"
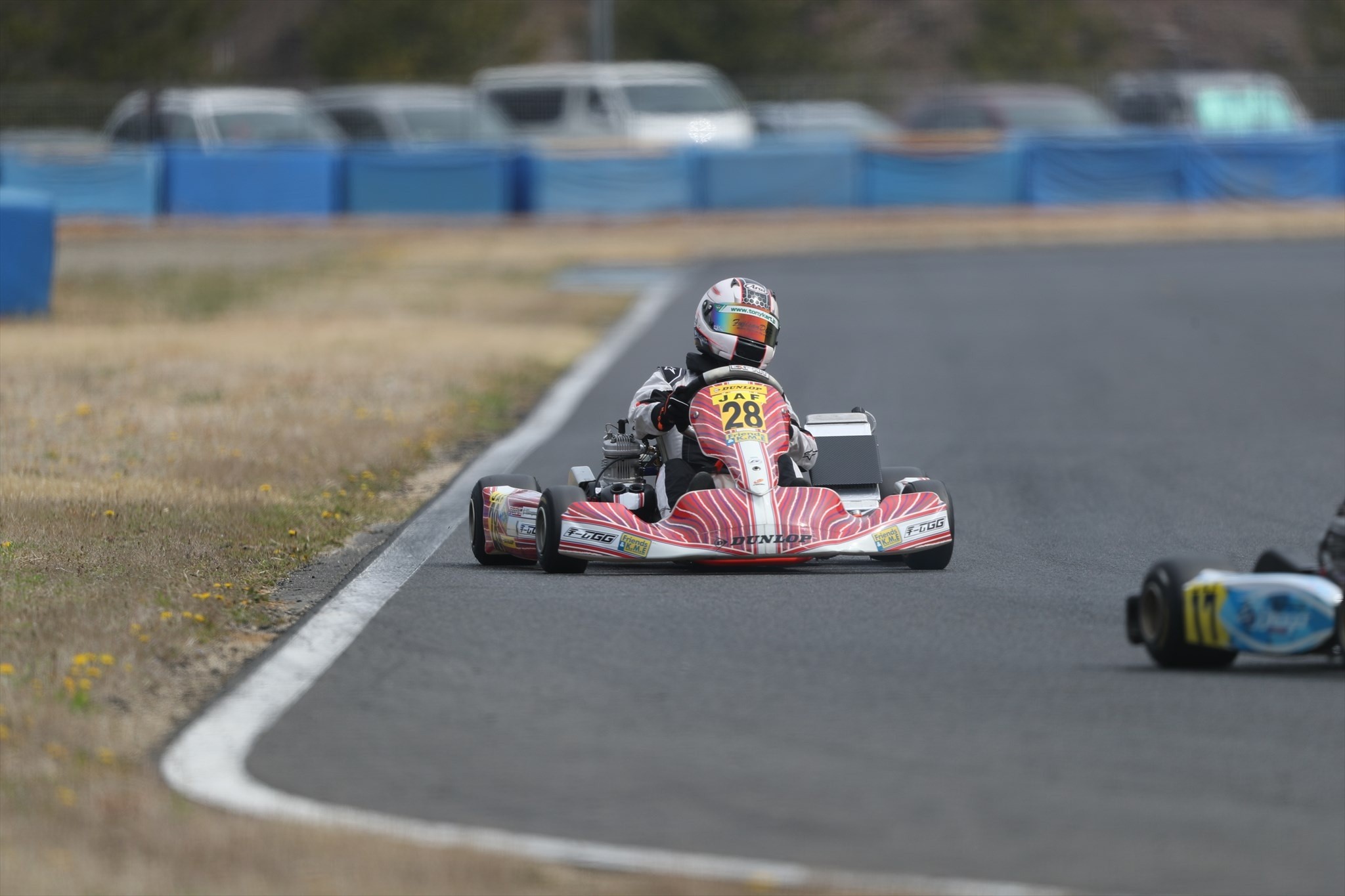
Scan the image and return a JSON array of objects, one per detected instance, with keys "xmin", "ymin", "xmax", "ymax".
[{"xmin": 627, "ymin": 276, "xmax": 818, "ymax": 522}]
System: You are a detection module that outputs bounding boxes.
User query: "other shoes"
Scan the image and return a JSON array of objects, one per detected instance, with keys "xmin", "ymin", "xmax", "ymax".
[
  {"xmin": 686, "ymin": 472, "xmax": 716, "ymax": 492},
  {"xmin": 790, "ymin": 478, "xmax": 811, "ymax": 487}
]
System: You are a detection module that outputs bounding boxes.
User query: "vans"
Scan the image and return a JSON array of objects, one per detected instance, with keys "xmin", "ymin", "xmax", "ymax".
[
  {"xmin": 1108, "ymin": 68, "xmax": 1317, "ymax": 137},
  {"xmin": 475, "ymin": 55, "xmax": 765, "ymax": 162}
]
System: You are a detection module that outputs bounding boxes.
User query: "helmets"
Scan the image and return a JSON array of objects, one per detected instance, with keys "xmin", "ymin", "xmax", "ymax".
[{"xmin": 692, "ymin": 277, "xmax": 779, "ymax": 369}]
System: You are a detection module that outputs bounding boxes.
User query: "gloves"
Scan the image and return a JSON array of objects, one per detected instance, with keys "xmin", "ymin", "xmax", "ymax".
[{"xmin": 650, "ymin": 385, "xmax": 691, "ymax": 432}]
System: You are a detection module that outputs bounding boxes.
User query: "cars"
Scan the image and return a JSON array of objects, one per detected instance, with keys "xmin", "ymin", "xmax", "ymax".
[
  {"xmin": 99, "ymin": 83, "xmax": 500, "ymax": 152},
  {"xmin": 754, "ymin": 66, "xmax": 1121, "ymax": 142}
]
